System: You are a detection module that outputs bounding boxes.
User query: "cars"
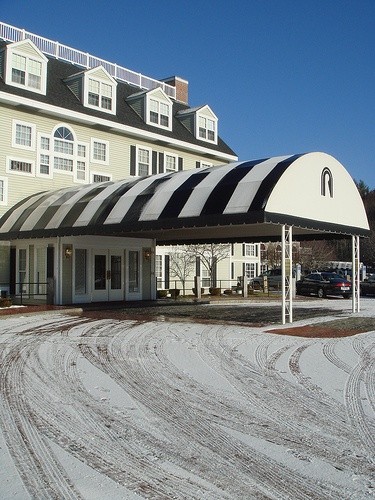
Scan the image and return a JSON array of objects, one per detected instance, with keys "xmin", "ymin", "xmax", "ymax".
[
  {"xmin": 358, "ymin": 272, "xmax": 375, "ymax": 296},
  {"xmin": 252, "ymin": 267, "xmax": 301, "ymax": 291},
  {"xmin": 296, "ymin": 272, "xmax": 358, "ymax": 298}
]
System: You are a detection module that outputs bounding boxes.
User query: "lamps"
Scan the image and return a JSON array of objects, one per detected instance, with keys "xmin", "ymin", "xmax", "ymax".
[
  {"xmin": 65, "ymin": 245, "xmax": 73, "ymax": 260},
  {"xmin": 144, "ymin": 247, "xmax": 152, "ymax": 257}
]
